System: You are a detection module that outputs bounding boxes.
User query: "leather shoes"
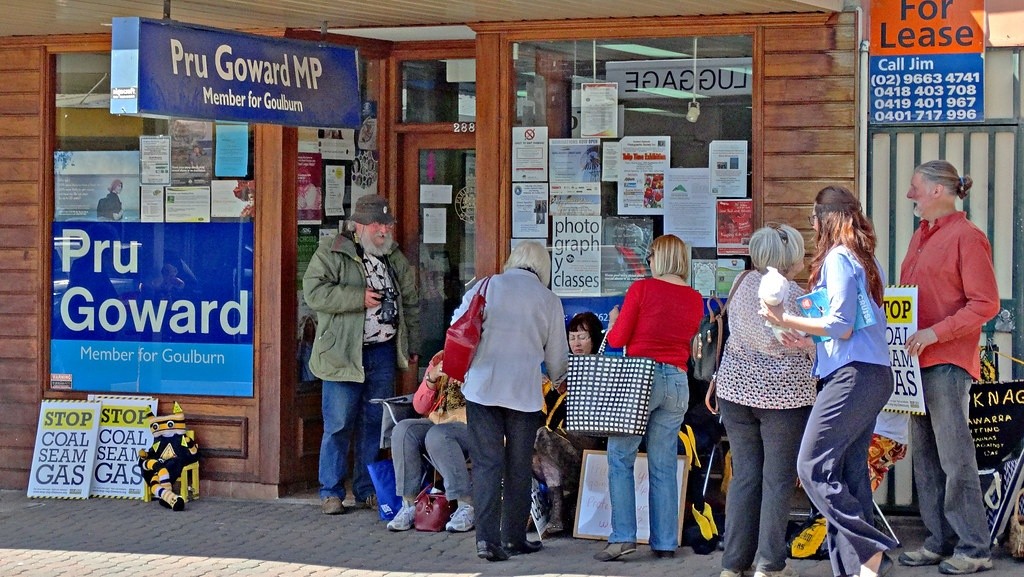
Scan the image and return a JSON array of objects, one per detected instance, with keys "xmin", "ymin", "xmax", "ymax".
[
  {"xmin": 475, "ymin": 541, "xmax": 512, "ymax": 562},
  {"xmin": 501, "ymin": 537, "xmax": 543, "ymax": 555}
]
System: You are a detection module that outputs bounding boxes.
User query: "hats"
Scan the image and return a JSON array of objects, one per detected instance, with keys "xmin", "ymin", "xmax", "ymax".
[{"xmin": 348, "ymin": 193, "xmax": 395, "ymax": 226}]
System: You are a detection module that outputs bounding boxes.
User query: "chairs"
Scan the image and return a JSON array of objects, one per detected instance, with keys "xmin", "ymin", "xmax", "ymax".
[
  {"xmin": 702, "ymin": 412, "xmax": 911, "ymax": 548},
  {"xmin": 144, "ymin": 430, "xmax": 200, "ymax": 503},
  {"xmin": 368, "ymin": 393, "xmax": 472, "ymax": 489}
]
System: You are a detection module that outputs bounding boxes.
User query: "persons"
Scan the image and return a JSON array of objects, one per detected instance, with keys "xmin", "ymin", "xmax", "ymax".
[
  {"xmin": 715, "ymin": 221, "xmax": 819, "ymax": 577},
  {"xmin": 303, "ymin": 194, "xmax": 424, "ymax": 515},
  {"xmin": 761, "ymin": 185, "xmax": 903, "ymax": 577},
  {"xmin": 594, "ymin": 234, "xmax": 705, "ymax": 561},
  {"xmin": 898, "ymin": 160, "xmax": 1000, "ymax": 575},
  {"xmin": 105, "ymin": 179, "xmax": 126, "ymax": 220},
  {"xmin": 534, "ymin": 312, "xmax": 609, "ymax": 539},
  {"xmin": 387, "ymin": 350, "xmax": 476, "ymax": 533},
  {"xmin": 450, "ymin": 240, "xmax": 569, "ymax": 562}
]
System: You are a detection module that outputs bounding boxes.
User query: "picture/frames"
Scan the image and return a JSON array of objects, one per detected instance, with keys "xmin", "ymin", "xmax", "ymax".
[{"xmin": 571, "ymin": 449, "xmax": 691, "ymax": 548}]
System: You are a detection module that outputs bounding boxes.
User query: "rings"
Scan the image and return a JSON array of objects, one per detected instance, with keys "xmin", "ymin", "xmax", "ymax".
[{"xmin": 917, "ymin": 343, "xmax": 920, "ymax": 346}]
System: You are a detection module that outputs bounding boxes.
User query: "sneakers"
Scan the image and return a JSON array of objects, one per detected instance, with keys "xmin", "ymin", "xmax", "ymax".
[
  {"xmin": 387, "ymin": 498, "xmax": 415, "ymax": 531},
  {"xmin": 445, "ymin": 504, "xmax": 476, "ymax": 533}
]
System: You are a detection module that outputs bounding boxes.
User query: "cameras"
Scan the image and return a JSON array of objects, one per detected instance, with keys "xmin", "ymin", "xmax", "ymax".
[{"xmin": 373, "ymin": 286, "xmax": 400, "ymax": 324}]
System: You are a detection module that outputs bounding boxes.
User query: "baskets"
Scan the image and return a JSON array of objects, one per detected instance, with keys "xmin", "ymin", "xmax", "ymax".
[{"xmin": 1009, "ymin": 490, "xmax": 1024, "ymax": 558}]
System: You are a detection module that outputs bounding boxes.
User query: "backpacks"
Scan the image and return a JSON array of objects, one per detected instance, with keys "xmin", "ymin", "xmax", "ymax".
[{"xmin": 691, "ymin": 271, "xmax": 750, "ymax": 414}]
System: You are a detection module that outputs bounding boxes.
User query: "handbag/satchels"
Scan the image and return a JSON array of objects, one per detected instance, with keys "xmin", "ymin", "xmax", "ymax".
[
  {"xmin": 415, "ymin": 481, "xmax": 450, "ymax": 532},
  {"xmin": 533, "ymin": 390, "xmax": 583, "ymax": 482},
  {"xmin": 565, "ymin": 328, "xmax": 656, "ymax": 438},
  {"xmin": 441, "ymin": 275, "xmax": 491, "ymax": 383}
]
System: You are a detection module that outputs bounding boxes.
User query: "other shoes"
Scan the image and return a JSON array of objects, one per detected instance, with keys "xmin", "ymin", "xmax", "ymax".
[
  {"xmin": 355, "ymin": 493, "xmax": 380, "ymax": 510},
  {"xmin": 719, "ymin": 564, "xmax": 757, "ymax": 577},
  {"xmin": 754, "ymin": 564, "xmax": 799, "ymax": 577},
  {"xmin": 939, "ymin": 550, "xmax": 993, "ymax": 574},
  {"xmin": 593, "ymin": 542, "xmax": 636, "ymax": 562},
  {"xmin": 654, "ymin": 550, "xmax": 676, "ymax": 559},
  {"xmin": 850, "ymin": 549, "xmax": 893, "ymax": 577},
  {"xmin": 898, "ymin": 546, "xmax": 946, "ymax": 567},
  {"xmin": 320, "ymin": 495, "xmax": 344, "ymax": 514}
]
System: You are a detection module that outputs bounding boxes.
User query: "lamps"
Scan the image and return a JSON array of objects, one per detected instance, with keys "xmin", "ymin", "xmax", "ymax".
[
  {"xmin": 55, "ymin": 72, "xmax": 110, "ymax": 109},
  {"xmin": 685, "ymin": 36, "xmax": 701, "ymax": 123}
]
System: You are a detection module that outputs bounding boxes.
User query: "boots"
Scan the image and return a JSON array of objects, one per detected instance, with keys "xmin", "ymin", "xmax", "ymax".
[{"xmin": 543, "ymin": 486, "xmax": 565, "ymax": 533}]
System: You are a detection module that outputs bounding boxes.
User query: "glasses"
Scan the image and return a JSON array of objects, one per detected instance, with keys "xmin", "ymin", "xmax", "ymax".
[
  {"xmin": 807, "ymin": 212, "xmax": 819, "ymax": 227},
  {"xmin": 645, "ymin": 252, "xmax": 655, "ymax": 267},
  {"xmin": 768, "ymin": 221, "xmax": 788, "ymax": 250}
]
